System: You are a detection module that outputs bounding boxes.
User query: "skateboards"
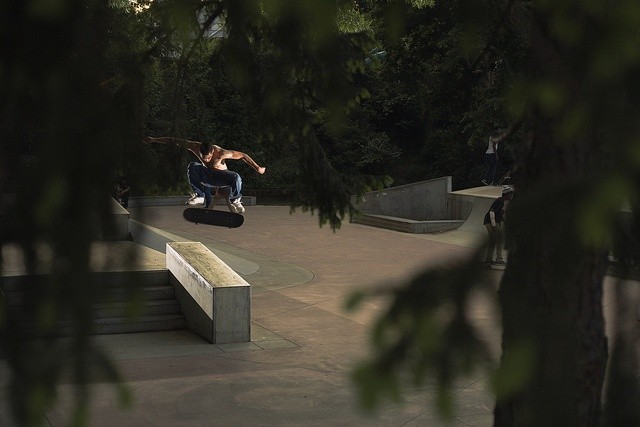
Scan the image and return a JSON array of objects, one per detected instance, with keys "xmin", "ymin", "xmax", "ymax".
[
  {"xmin": 182, "ymin": 207, "xmax": 244, "ymax": 229},
  {"xmin": 480, "ymin": 260, "xmax": 509, "ymax": 270}
]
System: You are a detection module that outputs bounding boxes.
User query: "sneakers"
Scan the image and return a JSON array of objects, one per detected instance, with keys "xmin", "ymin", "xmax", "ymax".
[
  {"xmin": 185, "ymin": 192, "xmax": 206, "ymax": 205},
  {"xmin": 496, "ymin": 256, "xmax": 504, "ymax": 264},
  {"xmin": 485, "ymin": 257, "xmax": 493, "ymax": 264},
  {"xmin": 233, "ymin": 195, "xmax": 246, "ymax": 214}
]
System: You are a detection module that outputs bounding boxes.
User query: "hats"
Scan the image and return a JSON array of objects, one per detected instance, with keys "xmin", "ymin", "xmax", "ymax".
[{"xmin": 502, "ymin": 185, "xmax": 513, "ymax": 197}]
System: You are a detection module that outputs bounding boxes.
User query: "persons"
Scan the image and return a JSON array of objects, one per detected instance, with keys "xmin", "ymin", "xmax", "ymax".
[
  {"xmin": 143, "ymin": 136, "xmax": 267, "ymax": 214},
  {"xmin": 113, "ymin": 167, "xmax": 130, "ymax": 209},
  {"xmin": 484, "ymin": 185, "xmax": 513, "ymax": 265},
  {"xmin": 481, "ymin": 130, "xmax": 504, "ymax": 186}
]
